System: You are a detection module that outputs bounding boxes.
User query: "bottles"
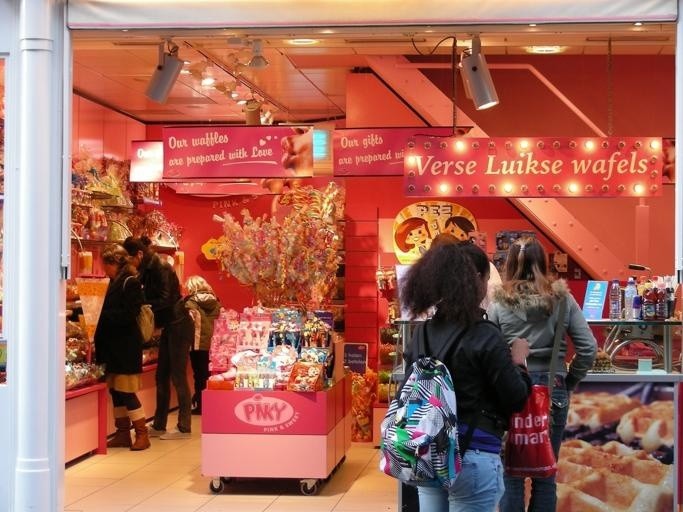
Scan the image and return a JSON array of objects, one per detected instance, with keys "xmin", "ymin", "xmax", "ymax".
[{"xmin": 608, "ymin": 275, "xmax": 675, "ymax": 321}]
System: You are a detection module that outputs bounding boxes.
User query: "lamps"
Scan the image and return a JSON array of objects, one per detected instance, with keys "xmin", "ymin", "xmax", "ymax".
[
  {"xmin": 457, "ymin": 34, "xmax": 499, "ymax": 111},
  {"xmin": 187, "ymin": 48, "xmax": 284, "ymax": 125},
  {"xmin": 247, "ymin": 40, "xmax": 269, "ymax": 69},
  {"xmin": 142, "ymin": 41, "xmax": 183, "ymax": 103}
]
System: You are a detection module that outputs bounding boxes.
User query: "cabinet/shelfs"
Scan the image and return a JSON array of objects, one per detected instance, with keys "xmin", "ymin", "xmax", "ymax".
[{"xmin": 66, "ymin": 189, "xmax": 196, "ymax": 467}]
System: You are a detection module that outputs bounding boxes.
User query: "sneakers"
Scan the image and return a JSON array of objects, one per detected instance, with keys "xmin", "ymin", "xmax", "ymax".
[
  {"xmin": 161, "ymin": 428, "xmax": 192, "ymax": 439},
  {"xmin": 191, "ymin": 408, "xmax": 201, "ymax": 415},
  {"xmin": 147, "ymin": 425, "xmax": 166, "ymax": 438}
]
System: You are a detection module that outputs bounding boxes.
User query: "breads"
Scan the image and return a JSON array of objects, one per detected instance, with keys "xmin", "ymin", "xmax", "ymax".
[{"xmin": 523, "ymin": 390, "xmax": 674, "ymax": 512}]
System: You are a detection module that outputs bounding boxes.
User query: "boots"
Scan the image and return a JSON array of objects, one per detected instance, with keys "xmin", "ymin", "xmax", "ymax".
[
  {"xmin": 132, "ymin": 417, "xmax": 151, "ymax": 451},
  {"xmin": 107, "ymin": 416, "xmax": 132, "ymax": 448}
]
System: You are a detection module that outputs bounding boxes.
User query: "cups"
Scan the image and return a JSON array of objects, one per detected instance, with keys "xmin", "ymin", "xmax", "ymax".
[
  {"xmin": 638, "ymin": 358, "xmax": 654, "ymax": 371},
  {"xmin": 392, "ymin": 334, "xmax": 401, "ymax": 343},
  {"xmin": 388, "ymin": 352, "xmax": 398, "ymax": 361}
]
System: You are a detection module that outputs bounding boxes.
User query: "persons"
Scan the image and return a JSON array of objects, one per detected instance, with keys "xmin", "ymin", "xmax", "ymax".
[
  {"xmin": 658, "ymin": 139, "xmax": 676, "ymax": 183},
  {"xmin": 93, "ymin": 242, "xmax": 151, "ymax": 451},
  {"xmin": 482, "ymin": 232, "xmax": 596, "ymax": 511},
  {"xmin": 442, "ymin": 214, "xmax": 477, "ymax": 244},
  {"xmin": 277, "ymin": 125, "xmax": 313, "ymax": 176},
  {"xmin": 258, "ymin": 177, "xmax": 291, "ymax": 194},
  {"xmin": 121, "ymin": 231, "xmax": 196, "ymax": 440},
  {"xmin": 182, "ymin": 275, "xmax": 220, "ymax": 415},
  {"xmin": 396, "ymin": 241, "xmax": 528, "ymax": 512},
  {"xmin": 394, "ymin": 216, "xmax": 432, "ymax": 256}
]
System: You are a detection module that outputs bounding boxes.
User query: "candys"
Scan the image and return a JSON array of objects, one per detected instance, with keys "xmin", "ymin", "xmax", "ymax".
[{"xmin": 203, "ymin": 181, "xmax": 344, "ymax": 389}]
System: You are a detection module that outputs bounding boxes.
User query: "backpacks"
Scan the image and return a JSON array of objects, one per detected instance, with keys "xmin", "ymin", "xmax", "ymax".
[
  {"xmin": 379, "ymin": 317, "xmax": 500, "ymax": 487},
  {"xmin": 123, "ymin": 276, "xmax": 155, "ymax": 343}
]
